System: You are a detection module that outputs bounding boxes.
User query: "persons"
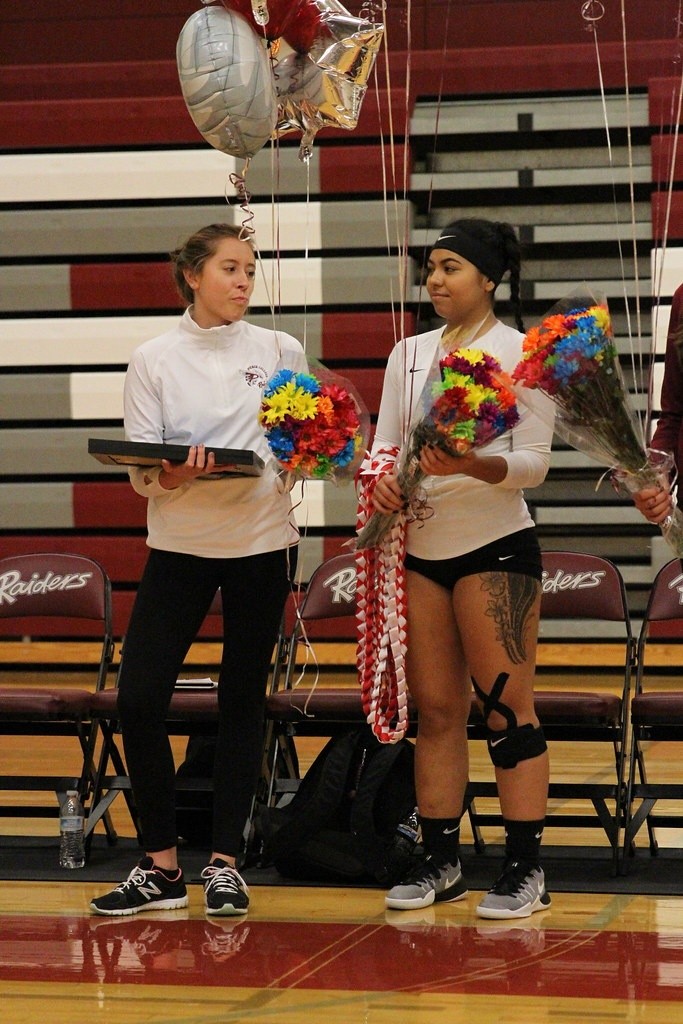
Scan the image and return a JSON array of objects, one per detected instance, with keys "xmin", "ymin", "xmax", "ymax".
[
  {"xmin": 88, "ymin": 226, "xmax": 309, "ymax": 920},
  {"xmin": 357, "ymin": 216, "xmax": 562, "ymax": 921},
  {"xmin": 633, "ymin": 285, "xmax": 683, "ymax": 527}
]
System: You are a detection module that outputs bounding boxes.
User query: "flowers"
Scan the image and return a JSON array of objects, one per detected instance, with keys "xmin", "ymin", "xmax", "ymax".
[
  {"xmin": 262, "ymin": 370, "xmax": 364, "ymax": 482},
  {"xmin": 356, "ymin": 347, "xmax": 527, "ymax": 549},
  {"xmin": 511, "ymin": 286, "xmax": 682, "ymax": 546}
]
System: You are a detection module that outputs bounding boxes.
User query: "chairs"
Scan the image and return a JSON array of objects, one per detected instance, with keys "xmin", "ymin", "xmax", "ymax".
[{"xmin": 2, "ymin": 547, "xmax": 681, "ymax": 882}]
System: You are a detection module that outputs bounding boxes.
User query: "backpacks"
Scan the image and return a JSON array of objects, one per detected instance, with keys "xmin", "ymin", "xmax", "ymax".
[
  {"xmin": 168, "ymin": 718, "xmax": 300, "ymax": 852},
  {"xmin": 261, "ymin": 726, "xmax": 422, "ymax": 883}
]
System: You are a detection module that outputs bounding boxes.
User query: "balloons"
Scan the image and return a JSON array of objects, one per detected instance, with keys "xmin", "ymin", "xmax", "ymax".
[{"xmin": 174, "ymin": 0, "xmax": 385, "ymax": 163}]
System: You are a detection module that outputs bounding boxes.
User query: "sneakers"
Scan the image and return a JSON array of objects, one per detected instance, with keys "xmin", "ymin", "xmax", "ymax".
[
  {"xmin": 385, "ymin": 850, "xmax": 468, "ymax": 910},
  {"xmin": 89, "ymin": 909, "xmax": 188, "ymax": 954},
  {"xmin": 478, "ymin": 912, "xmax": 550, "ymax": 954},
  {"xmin": 90, "ymin": 856, "xmax": 188, "ymax": 915},
  {"xmin": 385, "ymin": 900, "xmax": 471, "ymax": 938},
  {"xmin": 201, "ymin": 858, "xmax": 250, "ymax": 915},
  {"xmin": 201, "ymin": 916, "xmax": 249, "ymax": 959},
  {"xmin": 476, "ymin": 856, "xmax": 552, "ymax": 919}
]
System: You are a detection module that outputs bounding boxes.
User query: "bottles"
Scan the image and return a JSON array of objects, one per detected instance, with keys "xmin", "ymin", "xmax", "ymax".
[
  {"xmin": 58, "ymin": 790, "xmax": 87, "ymax": 869},
  {"xmin": 391, "ymin": 807, "xmax": 419, "ymax": 853}
]
